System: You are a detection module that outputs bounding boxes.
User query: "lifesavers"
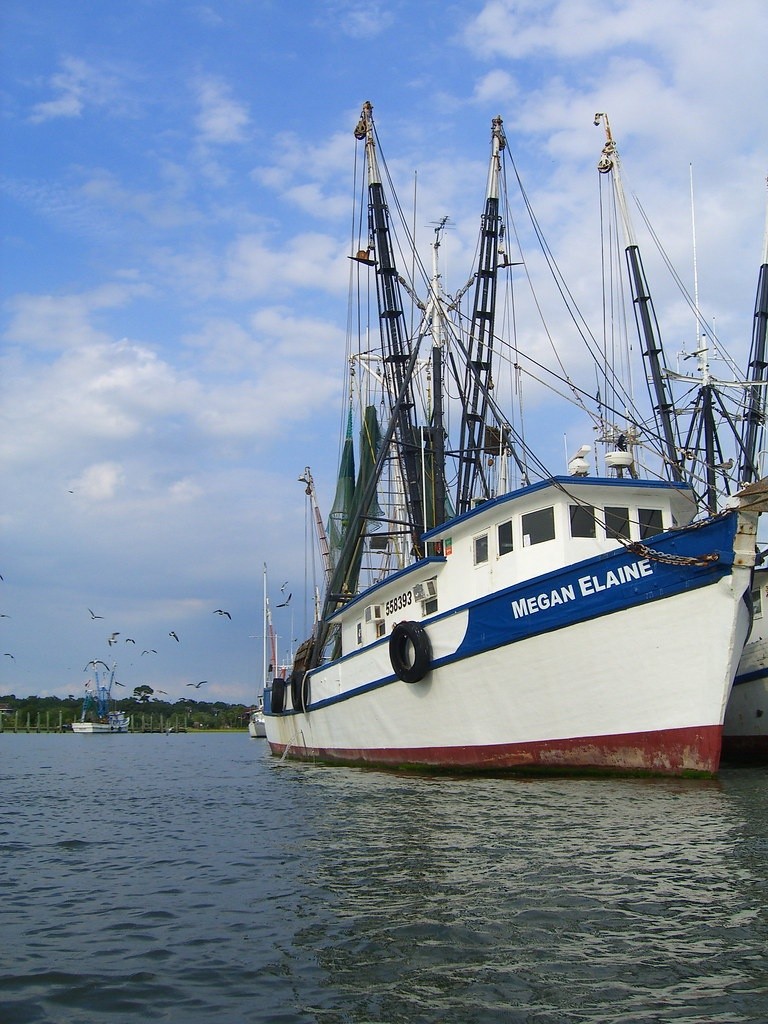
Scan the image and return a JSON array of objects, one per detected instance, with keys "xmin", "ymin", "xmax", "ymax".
[
  {"xmin": 271, "ymin": 678, "xmax": 286, "ymax": 714},
  {"xmin": 290, "ymin": 672, "xmax": 309, "ymax": 711},
  {"xmin": 388, "ymin": 621, "xmax": 433, "ymax": 684}
]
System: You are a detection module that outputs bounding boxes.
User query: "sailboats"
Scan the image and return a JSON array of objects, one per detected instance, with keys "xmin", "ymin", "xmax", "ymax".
[
  {"xmin": 247, "ymin": 695, "xmax": 267, "ymax": 737},
  {"xmin": 261, "ymin": 93, "xmax": 768, "ymax": 777},
  {"xmin": 70, "ymin": 660, "xmax": 131, "ymax": 734}
]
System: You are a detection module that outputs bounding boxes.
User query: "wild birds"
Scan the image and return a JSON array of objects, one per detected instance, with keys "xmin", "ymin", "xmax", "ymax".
[{"xmin": 0, "ymin": 479, "xmax": 296, "ymax": 739}]
